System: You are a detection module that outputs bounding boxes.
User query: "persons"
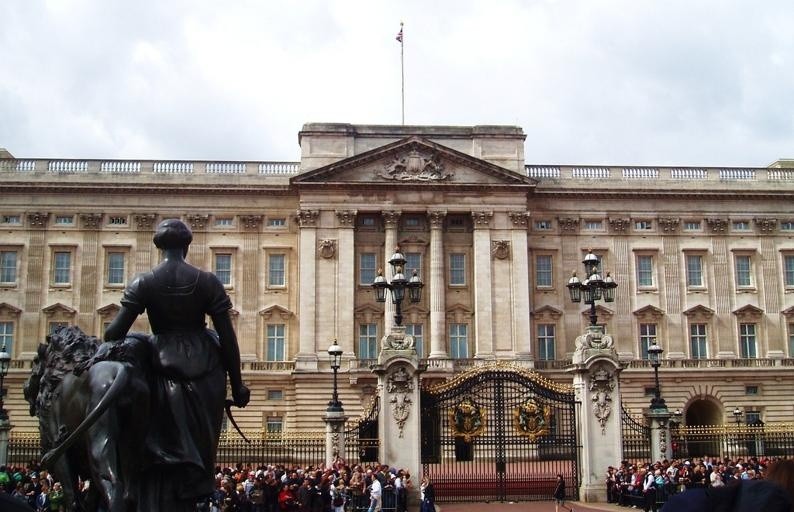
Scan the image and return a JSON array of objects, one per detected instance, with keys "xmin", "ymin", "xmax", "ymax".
[
  {"xmin": 98, "ymin": 218, "xmax": 249, "ymax": 509},
  {"xmin": 419, "ymin": 475, "xmax": 435, "ymax": 512},
  {"xmin": 211, "ymin": 455, "xmax": 412, "ymax": 512},
  {"xmin": 551, "ymin": 474, "xmax": 572, "ymax": 511},
  {"xmin": 0, "ymin": 464, "xmax": 64, "ymax": 512},
  {"xmin": 604, "ymin": 454, "xmax": 794, "ymax": 512}
]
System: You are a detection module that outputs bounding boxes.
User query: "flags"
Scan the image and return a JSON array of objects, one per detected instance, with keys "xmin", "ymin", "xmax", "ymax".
[{"xmin": 396, "ymin": 30, "xmax": 402, "ymax": 43}]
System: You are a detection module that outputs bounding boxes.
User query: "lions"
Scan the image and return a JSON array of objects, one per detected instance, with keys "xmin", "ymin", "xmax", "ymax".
[{"xmin": 23, "ymin": 323, "xmax": 162, "ymax": 512}]
